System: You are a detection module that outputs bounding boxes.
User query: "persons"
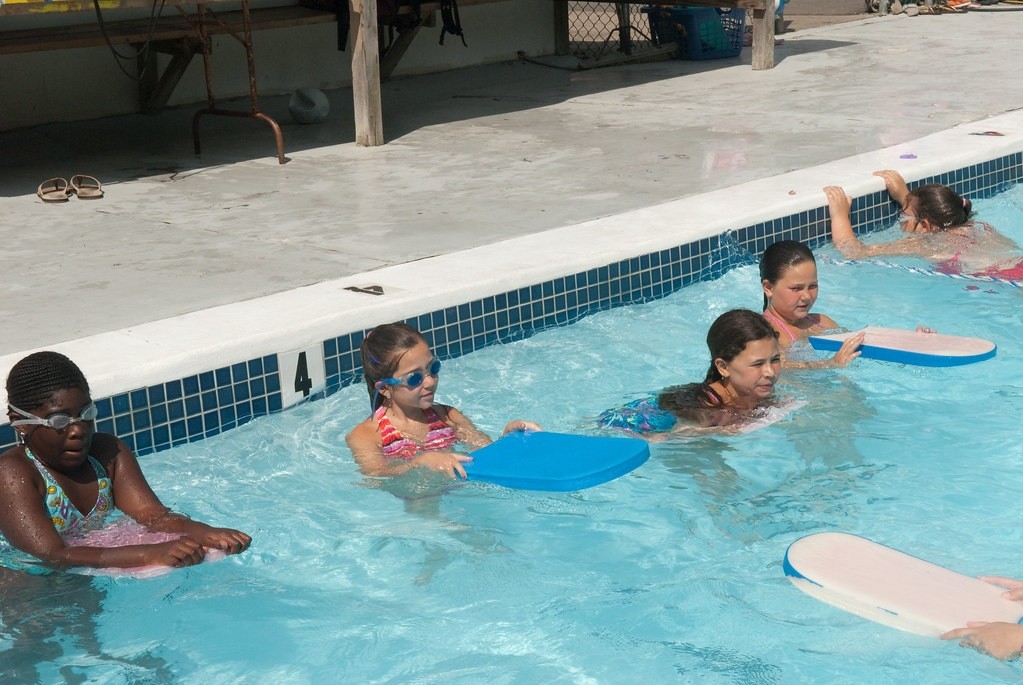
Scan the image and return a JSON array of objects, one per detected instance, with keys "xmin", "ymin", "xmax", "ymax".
[
  {"xmin": 344, "ymin": 324, "xmax": 546, "ymax": 480},
  {"xmin": 0, "ymin": 351, "xmax": 253, "ymax": 570},
  {"xmin": 598, "ymin": 308, "xmax": 782, "ymax": 443},
  {"xmin": 940, "ymin": 576, "xmax": 1023, "ymax": 661},
  {"xmin": 823, "ymin": 170, "xmax": 1023, "ymax": 287},
  {"xmin": 758, "ymin": 240, "xmax": 938, "ymax": 370}
]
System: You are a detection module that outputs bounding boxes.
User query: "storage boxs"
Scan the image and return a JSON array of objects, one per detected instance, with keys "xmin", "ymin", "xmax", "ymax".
[{"xmin": 640, "ymin": 4, "xmax": 748, "ymax": 61}]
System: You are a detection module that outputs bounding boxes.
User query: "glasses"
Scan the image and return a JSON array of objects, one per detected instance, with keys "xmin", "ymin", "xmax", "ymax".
[
  {"xmin": 5, "ymin": 403, "xmax": 103, "ymax": 431},
  {"xmin": 897, "ymin": 210, "xmax": 928, "ymax": 226},
  {"xmin": 376, "ymin": 353, "xmax": 440, "ymax": 388}
]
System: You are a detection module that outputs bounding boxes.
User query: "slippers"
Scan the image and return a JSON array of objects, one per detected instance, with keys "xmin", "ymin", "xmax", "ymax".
[
  {"xmin": 69, "ymin": 170, "xmax": 105, "ymax": 199},
  {"xmin": 37, "ymin": 175, "xmax": 70, "ymax": 202},
  {"xmin": 902, "ymin": 1, "xmax": 917, "ymax": 16}
]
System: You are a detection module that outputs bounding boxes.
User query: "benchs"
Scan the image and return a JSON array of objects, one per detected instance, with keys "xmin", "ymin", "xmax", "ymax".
[{"xmin": 1, "ymin": 2, "xmax": 462, "ymax": 116}]
